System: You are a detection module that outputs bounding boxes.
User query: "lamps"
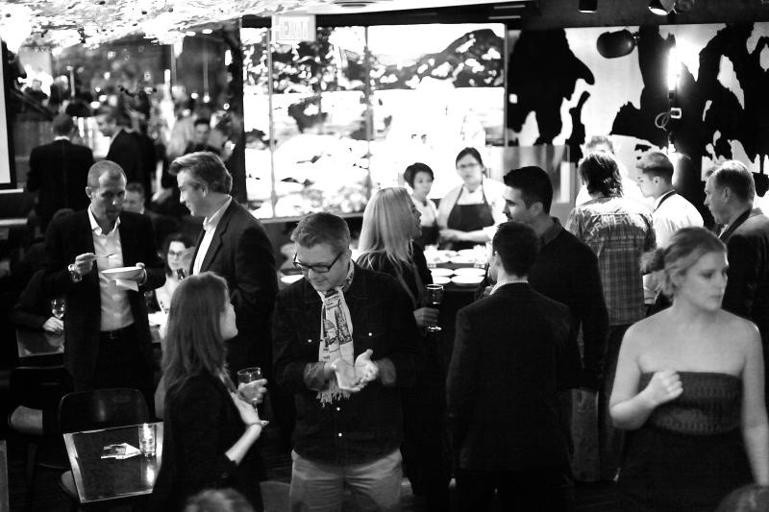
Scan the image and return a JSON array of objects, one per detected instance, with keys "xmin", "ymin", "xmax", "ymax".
[{"xmin": 648, "ymin": 0, "xmax": 675, "ymax": 15}]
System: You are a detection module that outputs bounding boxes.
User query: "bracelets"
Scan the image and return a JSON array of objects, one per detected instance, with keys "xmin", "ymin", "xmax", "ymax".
[
  {"xmin": 247, "ymin": 421, "xmax": 270, "ymax": 427},
  {"xmin": 68, "ymin": 264, "xmax": 81, "ymax": 281}
]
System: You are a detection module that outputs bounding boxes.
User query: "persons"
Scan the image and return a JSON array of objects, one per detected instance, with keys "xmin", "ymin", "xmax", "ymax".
[
  {"xmin": 19, "ymin": 209, "xmax": 78, "ymax": 335},
  {"xmin": 356, "ymin": 136, "xmax": 769, "ymax": 512},
  {"xmin": 48, "ymin": 159, "xmax": 171, "ymax": 395},
  {"xmin": 24, "ymin": 74, "xmax": 245, "ymax": 256},
  {"xmin": 271, "ymin": 212, "xmax": 430, "ymax": 512},
  {"xmin": 155, "ymin": 233, "xmax": 193, "ymax": 316},
  {"xmin": 169, "ymin": 151, "xmax": 279, "ymax": 483},
  {"xmin": 149, "ymin": 270, "xmax": 271, "ymax": 512}
]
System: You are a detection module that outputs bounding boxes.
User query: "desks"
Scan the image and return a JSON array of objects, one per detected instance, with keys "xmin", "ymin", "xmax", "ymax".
[
  {"xmin": 62, "ymin": 422, "xmax": 166, "ymax": 503},
  {"xmin": 14, "ymin": 321, "xmax": 161, "ymax": 365},
  {"xmin": 426, "ymin": 249, "xmax": 493, "ymax": 301}
]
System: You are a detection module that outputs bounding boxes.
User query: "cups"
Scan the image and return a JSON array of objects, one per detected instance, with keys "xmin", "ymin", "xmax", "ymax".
[{"xmin": 138, "ymin": 423, "xmax": 157, "ymax": 457}]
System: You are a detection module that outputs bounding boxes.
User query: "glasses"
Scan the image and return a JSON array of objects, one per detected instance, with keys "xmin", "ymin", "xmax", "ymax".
[{"xmin": 293, "ymin": 252, "xmax": 341, "ymax": 273}]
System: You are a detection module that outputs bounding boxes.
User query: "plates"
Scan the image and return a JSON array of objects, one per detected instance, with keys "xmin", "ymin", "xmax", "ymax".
[
  {"xmin": 100, "ymin": 266, "xmax": 143, "ymax": 280},
  {"xmin": 425, "ymin": 249, "xmax": 487, "ymax": 285}
]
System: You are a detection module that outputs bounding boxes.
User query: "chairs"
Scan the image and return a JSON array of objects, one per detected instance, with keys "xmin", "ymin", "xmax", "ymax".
[
  {"xmin": 57, "ymin": 387, "xmax": 150, "ymax": 496},
  {"xmin": 3, "ymin": 366, "xmax": 74, "ymax": 512}
]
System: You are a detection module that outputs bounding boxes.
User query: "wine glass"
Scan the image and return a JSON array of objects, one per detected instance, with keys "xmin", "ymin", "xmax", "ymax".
[
  {"xmin": 425, "ymin": 284, "xmax": 443, "ymax": 332},
  {"xmin": 51, "ymin": 298, "xmax": 65, "ymax": 319},
  {"xmin": 236, "ymin": 367, "xmax": 265, "ymax": 414}
]
